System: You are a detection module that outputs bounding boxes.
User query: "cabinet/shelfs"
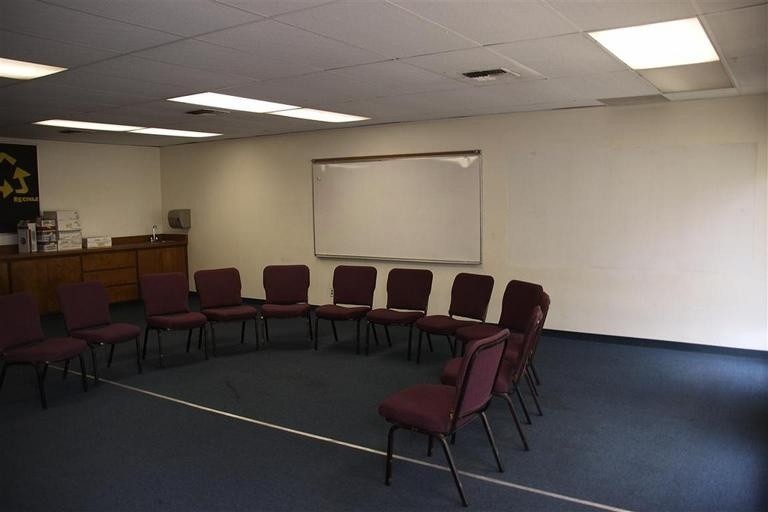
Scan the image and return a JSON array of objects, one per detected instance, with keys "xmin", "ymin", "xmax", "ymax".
[{"xmin": 0, "ymin": 234, "xmax": 189, "ymax": 314}]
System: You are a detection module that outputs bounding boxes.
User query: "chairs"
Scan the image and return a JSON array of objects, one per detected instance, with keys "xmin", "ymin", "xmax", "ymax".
[
  {"xmin": 140, "ymin": 272, "xmax": 209, "ymax": 368},
  {"xmin": 194, "ymin": 267, "xmax": 259, "ymax": 355},
  {"xmin": 428, "ymin": 312, "xmax": 544, "ymax": 469},
  {"xmin": 465, "ymin": 305, "xmax": 543, "ymax": 423},
  {"xmin": 454, "ymin": 280, "xmax": 542, "ymax": 358},
  {"xmin": 416, "ymin": 272, "xmax": 494, "ymax": 364},
  {"xmin": 57, "ymin": 283, "xmax": 144, "ymax": 381},
  {"xmin": 379, "ymin": 330, "xmax": 508, "ymax": 506},
  {"xmin": 314, "ymin": 264, "xmax": 379, "ymax": 355},
  {"xmin": 366, "ymin": 267, "xmax": 433, "ymax": 361},
  {"xmin": 0, "ymin": 291, "xmax": 88, "ymax": 408},
  {"xmin": 462, "ymin": 290, "xmax": 551, "ymax": 396},
  {"xmin": 261, "ymin": 264, "xmax": 314, "ymax": 340}
]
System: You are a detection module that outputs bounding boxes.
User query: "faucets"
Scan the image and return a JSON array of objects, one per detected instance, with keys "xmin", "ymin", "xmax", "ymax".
[{"xmin": 150, "ymin": 226, "xmax": 159, "ymax": 241}]
